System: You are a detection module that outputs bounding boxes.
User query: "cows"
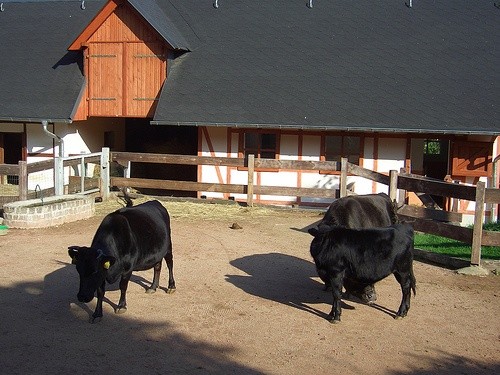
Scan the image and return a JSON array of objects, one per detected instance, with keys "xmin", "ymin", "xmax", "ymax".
[
  {"xmin": 309, "ymin": 191, "xmax": 419, "ymax": 325},
  {"xmin": 66, "ymin": 186, "xmax": 178, "ymax": 325}
]
